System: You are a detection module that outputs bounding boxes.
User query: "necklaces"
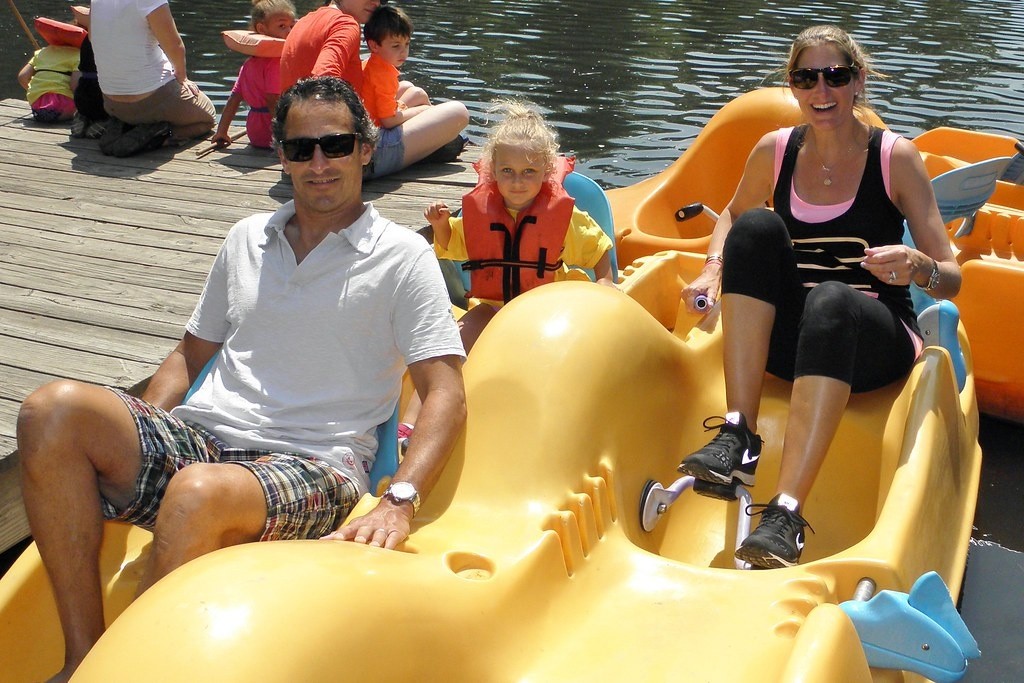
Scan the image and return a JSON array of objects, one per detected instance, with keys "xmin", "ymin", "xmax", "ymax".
[{"xmin": 815, "ymin": 137, "xmax": 852, "ymax": 186}]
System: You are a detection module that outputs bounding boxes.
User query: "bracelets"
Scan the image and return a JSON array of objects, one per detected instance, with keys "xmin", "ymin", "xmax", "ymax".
[{"xmin": 705, "ymin": 255, "xmax": 724, "ymax": 269}]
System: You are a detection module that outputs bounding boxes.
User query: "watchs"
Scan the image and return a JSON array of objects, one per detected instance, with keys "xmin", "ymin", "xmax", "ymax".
[
  {"xmin": 381, "ymin": 482, "xmax": 421, "ymax": 518},
  {"xmin": 914, "ymin": 260, "xmax": 940, "ymax": 291}
]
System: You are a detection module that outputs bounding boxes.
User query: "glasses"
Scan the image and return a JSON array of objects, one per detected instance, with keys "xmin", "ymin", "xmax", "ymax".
[
  {"xmin": 379, "ymin": 0, "xmax": 389, "ymax": 5},
  {"xmin": 276, "ymin": 132, "xmax": 362, "ymax": 163},
  {"xmin": 786, "ymin": 64, "xmax": 858, "ymax": 91}
]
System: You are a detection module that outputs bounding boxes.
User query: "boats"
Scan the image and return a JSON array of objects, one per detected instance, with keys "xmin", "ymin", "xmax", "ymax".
[
  {"xmin": 0, "ymin": 250, "xmax": 986, "ymax": 682},
  {"xmin": 605, "ymin": 85, "xmax": 1024, "ymax": 435}
]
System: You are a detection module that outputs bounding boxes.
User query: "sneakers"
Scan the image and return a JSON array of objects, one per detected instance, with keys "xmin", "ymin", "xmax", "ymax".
[
  {"xmin": 734, "ymin": 493, "xmax": 816, "ymax": 568},
  {"xmin": 674, "ymin": 411, "xmax": 763, "ymax": 487}
]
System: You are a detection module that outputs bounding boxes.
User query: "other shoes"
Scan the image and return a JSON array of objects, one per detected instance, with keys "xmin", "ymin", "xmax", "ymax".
[
  {"xmin": 84, "ymin": 116, "xmax": 117, "ymax": 140},
  {"xmin": 98, "ymin": 120, "xmax": 132, "ymax": 156},
  {"xmin": 69, "ymin": 110, "xmax": 87, "ymax": 138},
  {"xmin": 419, "ymin": 134, "xmax": 464, "ymax": 165},
  {"xmin": 112, "ymin": 122, "xmax": 173, "ymax": 156}
]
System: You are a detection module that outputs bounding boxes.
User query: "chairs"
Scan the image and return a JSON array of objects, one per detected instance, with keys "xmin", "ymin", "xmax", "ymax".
[
  {"xmin": 929, "ymin": 157, "xmax": 1013, "ymax": 238},
  {"xmin": 900, "ymin": 219, "xmax": 937, "ymax": 316},
  {"xmin": 452, "ymin": 170, "xmax": 617, "ymax": 293},
  {"xmin": 180, "ymin": 338, "xmax": 398, "ymax": 496}
]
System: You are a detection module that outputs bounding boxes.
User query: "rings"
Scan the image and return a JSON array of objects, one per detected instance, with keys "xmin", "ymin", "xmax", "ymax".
[
  {"xmin": 375, "ymin": 529, "xmax": 385, "ymax": 532},
  {"xmin": 889, "ymin": 271, "xmax": 896, "ymax": 284}
]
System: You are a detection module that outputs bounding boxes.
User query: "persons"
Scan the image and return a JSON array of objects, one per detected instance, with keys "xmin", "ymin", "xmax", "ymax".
[
  {"xmin": 362, "ymin": 5, "xmax": 431, "ymax": 129},
  {"xmin": 17, "ymin": 44, "xmax": 83, "ymax": 125},
  {"xmin": 280, "ymin": 0, "xmax": 470, "ymax": 180},
  {"xmin": 399, "ymin": 103, "xmax": 616, "ymax": 450},
  {"xmin": 211, "ymin": 0, "xmax": 297, "ymax": 148},
  {"xmin": 91, "ymin": 0, "xmax": 216, "ymax": 157},
  {"xmin": 679, "ymin": 26, "xmax": 962, "ymax": 570},
  {"xmin": 71, "ymin": 31, "xmax": 111, "ymax": 138},
  {"xmin": 16, "ymin": 75, "xmax": 467, "ymax": 683}
]
